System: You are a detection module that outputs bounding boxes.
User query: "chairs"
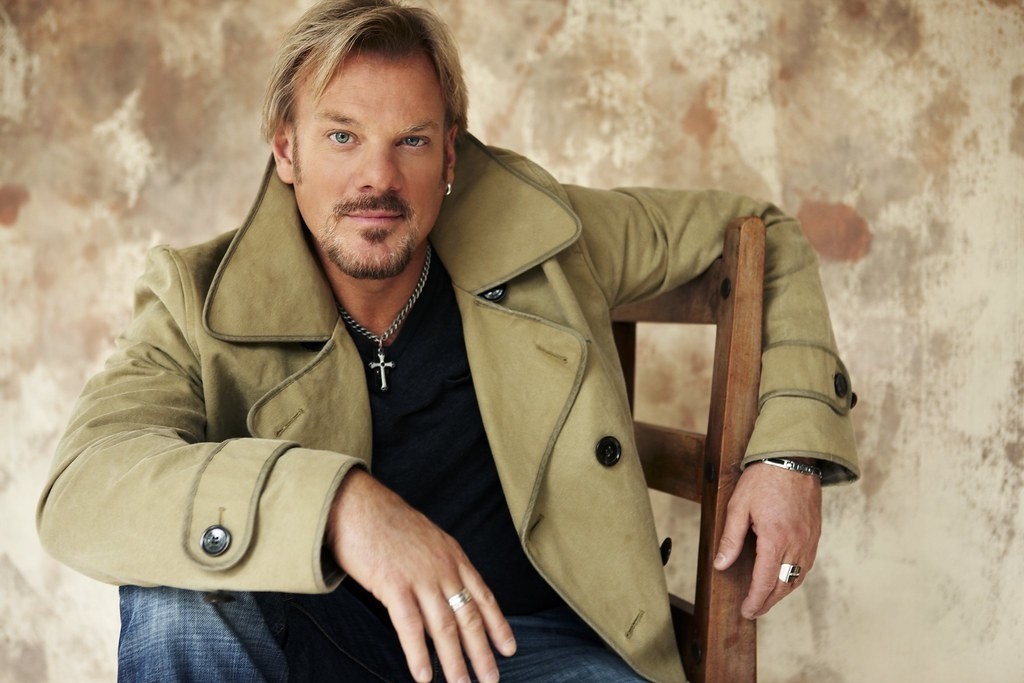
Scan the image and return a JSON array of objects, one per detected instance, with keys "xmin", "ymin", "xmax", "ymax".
[{"xmin": 608, "ymin": 215, "xmax": 768, "ymax": 683}]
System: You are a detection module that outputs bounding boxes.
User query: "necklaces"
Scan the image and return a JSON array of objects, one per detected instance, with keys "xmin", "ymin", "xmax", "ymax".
[{"xmin": 313, "ymin": 238, "xmax": 432, "ymax": 394}]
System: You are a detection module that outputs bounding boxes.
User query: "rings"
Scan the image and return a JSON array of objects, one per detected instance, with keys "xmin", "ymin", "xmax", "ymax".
[
  {"xmin": 778, "ymin": 561, "xmax": 802, "ymax": 586},
  {"xmin": 448, "ymin": 586, "xmax": 473, "ymax": 611}
]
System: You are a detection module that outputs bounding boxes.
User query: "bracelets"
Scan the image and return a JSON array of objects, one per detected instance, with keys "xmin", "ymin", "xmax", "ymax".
[{"xmin": 761, "ymin": 457, "xmax": 823, "ymax": 483}]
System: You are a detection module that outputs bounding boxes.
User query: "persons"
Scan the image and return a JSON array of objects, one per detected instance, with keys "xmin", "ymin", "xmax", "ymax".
[{"xmin": 33, "ymin": 2, "xmax": 870, "ymax": 683}]
